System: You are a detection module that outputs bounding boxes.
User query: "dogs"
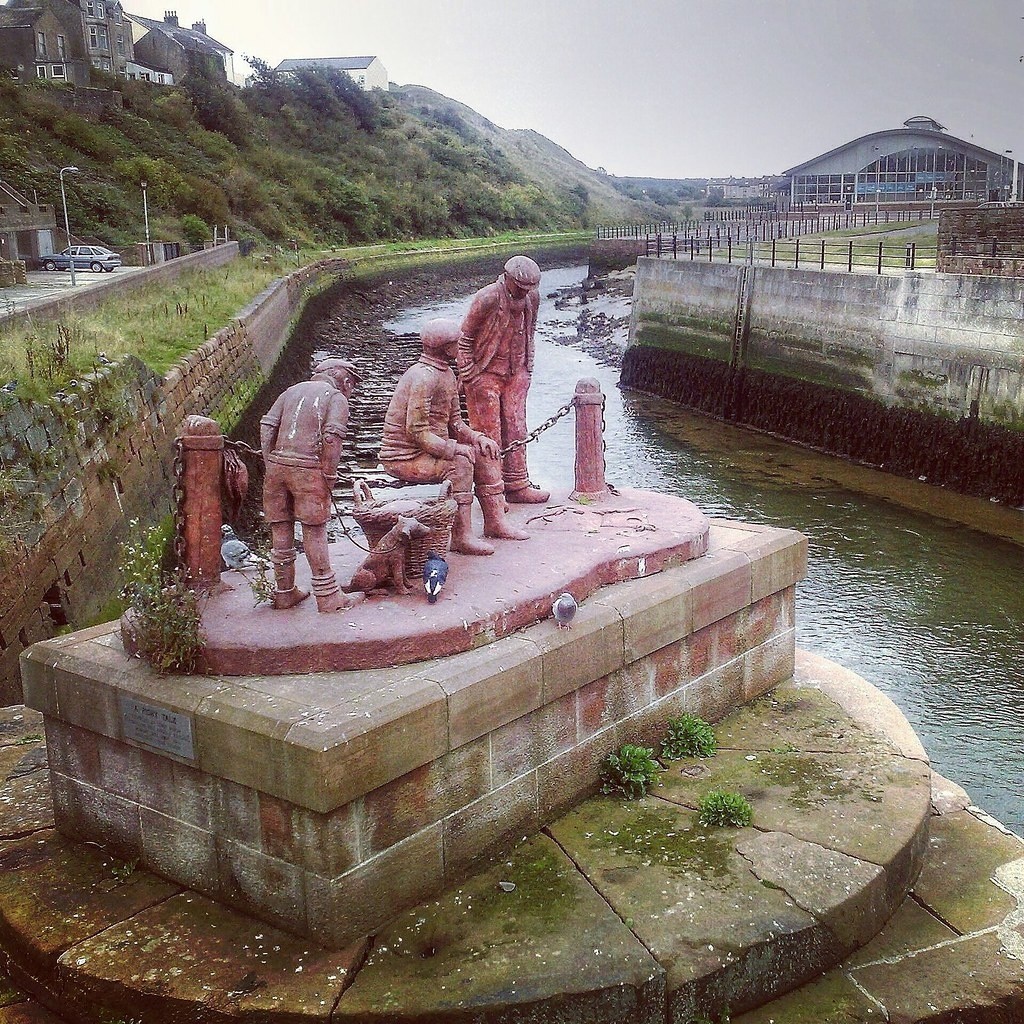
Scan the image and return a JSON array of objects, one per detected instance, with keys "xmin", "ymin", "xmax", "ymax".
[{"xmin": 340, "ymin": 514, "xmax": 431, "ymax": 596}]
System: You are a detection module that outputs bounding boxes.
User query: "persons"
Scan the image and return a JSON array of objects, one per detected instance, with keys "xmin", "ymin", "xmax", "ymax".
[
  {"xmin": 378, "ymin": 320, "xmax": 530, "ymax": 556},
  {"xmin": 259, "ymin": 358, "xmax": 366, "ymax": 613},
  {"xmin": 454, "ymin": 255, "xmax": 550, "ymax": 513}
]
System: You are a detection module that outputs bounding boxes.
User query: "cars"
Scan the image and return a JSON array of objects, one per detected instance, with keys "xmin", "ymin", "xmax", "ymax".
[{"xmin": 37, "ymin": 245, "xmax": 121, "ymax": 273}]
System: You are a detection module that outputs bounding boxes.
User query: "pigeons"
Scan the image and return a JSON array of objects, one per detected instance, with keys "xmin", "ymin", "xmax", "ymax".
[
  {"xmin": 220, "ymin": 524, "xmax": 270, "ymax": 572},
  {"xmin": 422, "ymin": 550, "xmax": 449, "ymax": 605},
  {"xmin": 553, "ymin": 593, "xmax": 577, "ymax": 632}
]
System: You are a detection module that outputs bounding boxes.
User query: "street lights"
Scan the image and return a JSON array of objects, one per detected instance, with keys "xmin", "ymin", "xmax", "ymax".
[
  {"xmin": 140, "ymin": 180, "xmax": 152, "ymax": 267},
  {"xmin": 60, "ymin": 166, "xmax": 78, "ymax": 286}
]
